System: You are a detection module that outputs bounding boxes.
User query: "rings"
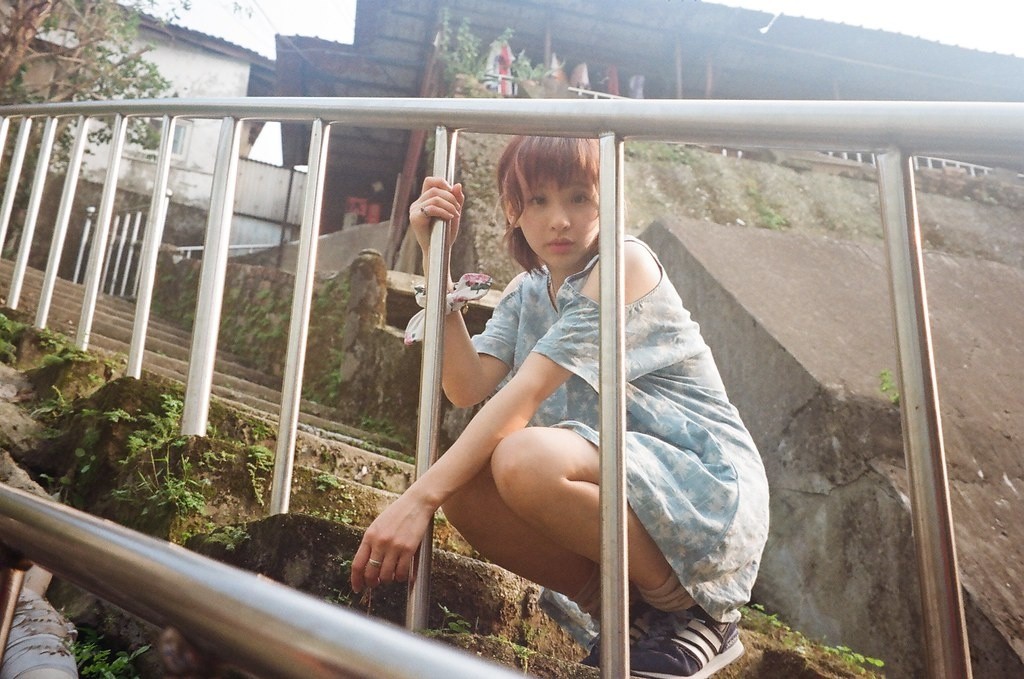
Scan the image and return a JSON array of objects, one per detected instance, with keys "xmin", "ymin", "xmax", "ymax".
[
  {"xmin": 421, "ymin": 208, "xmax": 431, "ymax": 218},
  {"xmin": 369, "ymin": 559, "xmax": 381, "ymax": 567}
]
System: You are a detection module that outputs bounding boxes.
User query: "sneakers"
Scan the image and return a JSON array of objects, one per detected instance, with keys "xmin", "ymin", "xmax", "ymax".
[
  {"xmin": 630, "ymin": 603, "xmax": 745, "ymax": 679},
  {"xmin": 580, "ymin": 604, "xmax": 661, "ymax": 667}
]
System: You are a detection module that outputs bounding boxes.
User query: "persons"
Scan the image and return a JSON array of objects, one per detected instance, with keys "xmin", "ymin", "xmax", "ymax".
[{"xmin": 351, "ymin": 134, "xmax": 768, "ymax": 679}]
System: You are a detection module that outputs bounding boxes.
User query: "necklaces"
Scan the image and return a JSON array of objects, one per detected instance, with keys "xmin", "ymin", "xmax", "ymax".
[{"xmin": 550, "ymin": 275, "xmax": 556, "ymax": 297}]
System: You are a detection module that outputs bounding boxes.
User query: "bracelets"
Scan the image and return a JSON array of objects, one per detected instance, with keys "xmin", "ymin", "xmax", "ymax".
[{"xmin": 404, "ymin": 273, "xmax": 493, "ymax": 345}]
